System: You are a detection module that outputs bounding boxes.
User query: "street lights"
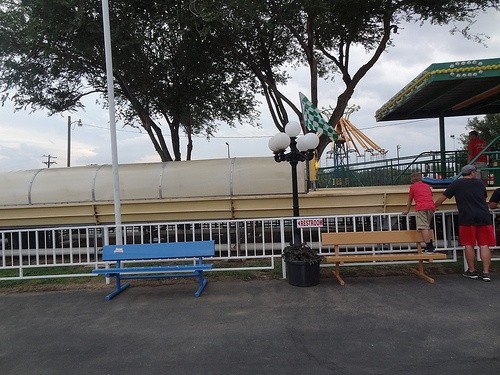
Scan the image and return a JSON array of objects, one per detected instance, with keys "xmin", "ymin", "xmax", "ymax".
[
  {"xmin": 225, "ymin": 142, "xmax": 230, "ymax": 158},
  {"xmin": 67, "ymin": 115, "xmax": 82, "ymax": 166},
  {"xmin": 268, "ymin": 121, "xmax": 319, "ymax": 248}
]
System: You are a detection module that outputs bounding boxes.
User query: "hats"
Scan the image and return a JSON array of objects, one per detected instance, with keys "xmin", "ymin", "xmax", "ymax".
[{"xmin": 461, "ymin": 165, "xmax": 481, "ymax": 174}]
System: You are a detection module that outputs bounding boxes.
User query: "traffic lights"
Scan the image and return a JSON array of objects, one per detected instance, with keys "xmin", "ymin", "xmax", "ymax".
[{"xmin": 309, "ymin": 151, "xmax": 318, "ymax": 181}]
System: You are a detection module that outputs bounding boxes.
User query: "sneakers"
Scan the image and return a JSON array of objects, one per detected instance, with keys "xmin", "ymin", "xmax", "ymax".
[
  {"xmin": 480, "ymin": 271, "xmax": 491, "ymax": 281},
  {"xmin": 463, "ymin": 268, "xmax": 478, "ymax": 279}
]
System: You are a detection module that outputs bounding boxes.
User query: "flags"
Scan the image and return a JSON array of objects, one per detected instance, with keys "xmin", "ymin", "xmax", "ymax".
[{"xmin": 299, "ymin": 92, "xmax": 340, "ymax": 139}]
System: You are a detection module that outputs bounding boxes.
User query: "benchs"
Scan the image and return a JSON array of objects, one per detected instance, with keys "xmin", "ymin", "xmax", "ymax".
[
  {"xmin": 92, "ymin": 240, "xmax": 215, "ymax": 302},
  {"xmin": 319, "ymin": 230, "xmax": 447, "ymax": 287}
]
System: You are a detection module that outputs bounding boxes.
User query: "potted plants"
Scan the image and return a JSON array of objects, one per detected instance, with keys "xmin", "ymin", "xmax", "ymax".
[{"xmin": 281, "ymin": 241, "xmax": 328, "ymax": 288}]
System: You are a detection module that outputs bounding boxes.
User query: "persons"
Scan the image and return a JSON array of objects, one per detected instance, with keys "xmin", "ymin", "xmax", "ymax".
[
  {"xmin": 436, "ymin": 165, "xmax": 497, "ymax": 281},
  {"xmin": 489, "ymin": 188, "xmax": 500, "ymax": 209},
  {"xmin": 402, "ymin": 171, "xmax": 436, "ymax": 254},
  {"xmin": 467, "ymin": 130, "xmax": 488, "ymax": 186}
]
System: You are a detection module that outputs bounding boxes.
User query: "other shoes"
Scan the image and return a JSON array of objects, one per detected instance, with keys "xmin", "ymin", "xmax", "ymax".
[{"xmin": 423, "ymin": 247, "xmax": 434, "ymax": 255}]
